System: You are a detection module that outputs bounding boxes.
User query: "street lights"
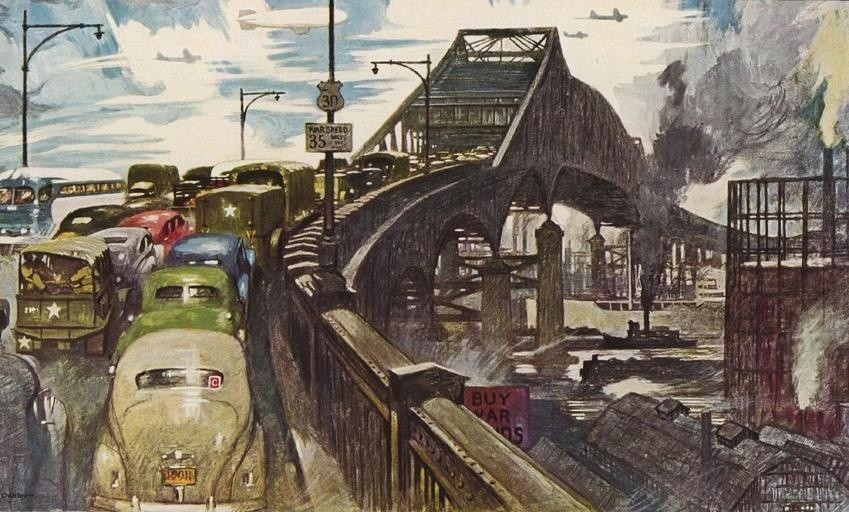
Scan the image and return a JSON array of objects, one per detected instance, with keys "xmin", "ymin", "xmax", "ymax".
[
  {"xmin": 240, "ymin": 88, "xmax": 285, "ymax": 160},
  {"xmin": 372, "ymin": 54, "xmax": 431, "ymax": 170},
  {"xmin": 22, "ymin": 9, "xmax": 106, "ymax": 167}
]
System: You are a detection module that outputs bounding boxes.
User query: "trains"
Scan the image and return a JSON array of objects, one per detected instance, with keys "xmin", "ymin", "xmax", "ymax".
[{"xmin": 580, "ymin": 354, "xmax": 682, "ymax": 383}]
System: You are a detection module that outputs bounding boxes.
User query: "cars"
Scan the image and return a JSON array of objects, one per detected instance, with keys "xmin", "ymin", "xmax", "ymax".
[{"xmin": 0, "ymin": 144, "xmax": 493, "ymax": 512}]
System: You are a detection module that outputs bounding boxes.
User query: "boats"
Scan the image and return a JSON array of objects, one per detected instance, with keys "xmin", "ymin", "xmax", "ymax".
[{"xmin": 510, "ymin": 311, "xmax": 699, "ymax": 349}]
